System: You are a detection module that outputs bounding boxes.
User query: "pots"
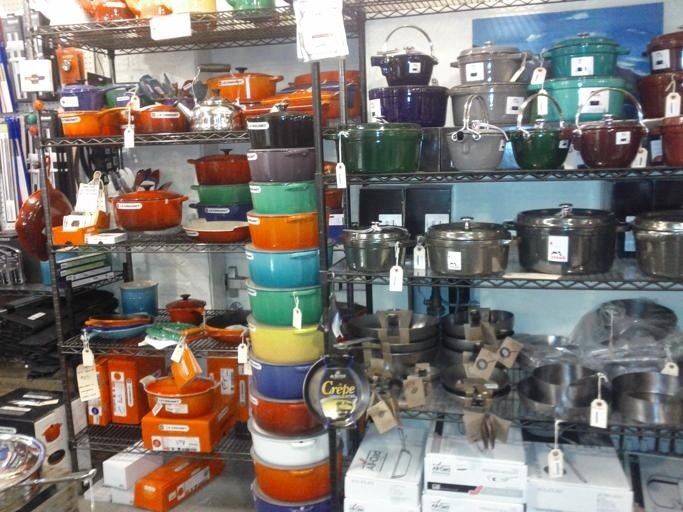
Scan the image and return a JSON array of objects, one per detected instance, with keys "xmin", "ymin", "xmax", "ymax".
[
  {"xmin": 504, "ymin": 203, "xmax": 617, "ymax": 276},
  {"xmin": 343, "ymin": 218, "xmax": 409, "ymax": 273},
  {"xmin": 302, "ymin": 294, "xmax": 682, "ymax": 430},
  {"xmin": 0, "ymin": 0, "xmax": 336, "ymax": 512},
  {"xmin": 616, "ymin": 207, "xmax": 682, "ymax": 279},
  {"xmin": 323, "ymin": 22, "xmax": 683, "ymax": 170},
  {"xmin": 422, "ymin": 216, "xmax": 515, "ymax": 277}
]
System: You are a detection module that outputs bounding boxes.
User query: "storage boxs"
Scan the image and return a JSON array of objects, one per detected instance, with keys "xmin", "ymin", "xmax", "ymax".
[
  {"xmin": 86, "ymin": 339, "xmax": 249, "ymax": 453},
  {"xmin": 0, "ymin": 387, "xmax": 88, "ymax": 473},
  {"xmin": 343, "ymin": 414, "xmax": 634, "ymax": 510}
]
